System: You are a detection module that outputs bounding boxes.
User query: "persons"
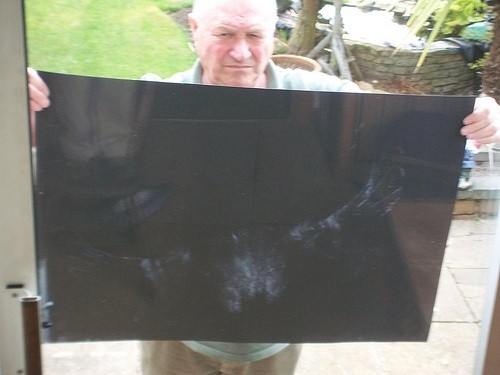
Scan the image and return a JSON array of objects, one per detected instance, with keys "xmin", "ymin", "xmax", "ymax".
[{"xmin": 28, "ymin": 0, "xmax": 500, "ymax": 374}]
